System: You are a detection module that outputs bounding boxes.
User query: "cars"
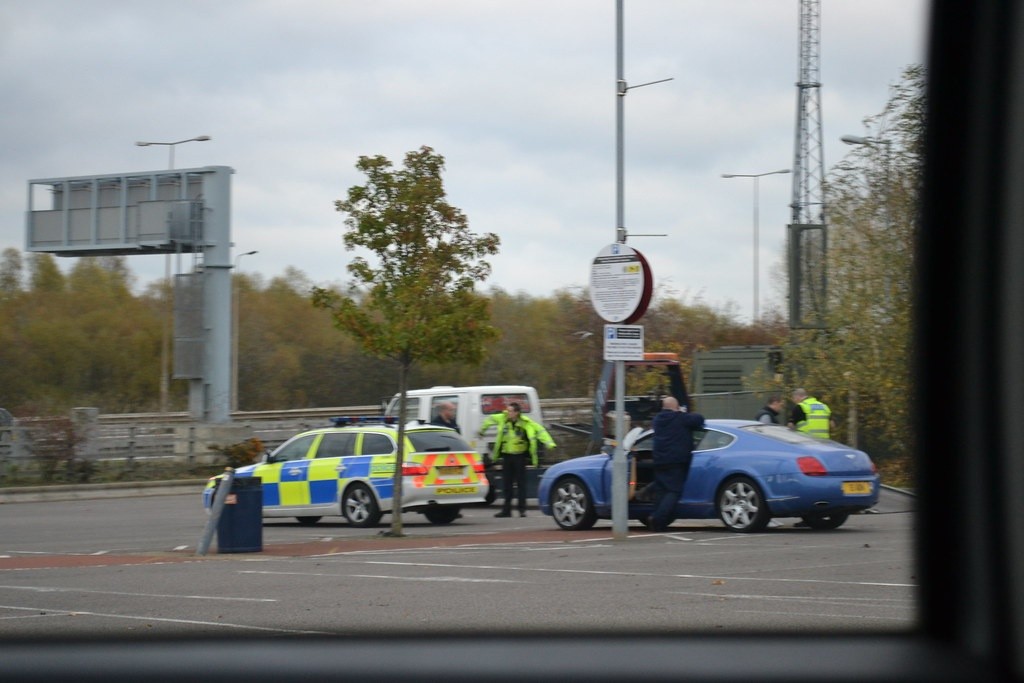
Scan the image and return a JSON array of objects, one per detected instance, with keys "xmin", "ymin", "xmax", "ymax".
[
  {"xmin": 536, "ymin": 414, "xmax": 881, "ymax": 534},
  {"xmin": 202, "ymin": 416, "xmax": 491, "ymax": 530}
]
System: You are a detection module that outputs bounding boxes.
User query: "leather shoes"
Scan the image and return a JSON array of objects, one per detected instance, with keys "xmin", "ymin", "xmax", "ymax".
[{"xmin": 793, "ymin": 521, "xmax": 808, "ymax": 527}]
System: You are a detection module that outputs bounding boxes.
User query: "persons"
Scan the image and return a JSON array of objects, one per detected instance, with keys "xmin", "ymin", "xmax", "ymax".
[
  {"xmin": 432, "ymin": 401, "xmax": 463, "ymax": 519},
  {"xmin": 788, "ymin": 388, "xmax": 835, "ymax": 527},
  {"xmin": 479, "ymin": 402, "xmax": 557, "ymax": 518},
  {"xmin": 755, "ymin": 396, "xmax": 784, "ymax": 528},
  {"xmin": 644, "ymin": 397, "xmax": 704, "ymax": 530}
]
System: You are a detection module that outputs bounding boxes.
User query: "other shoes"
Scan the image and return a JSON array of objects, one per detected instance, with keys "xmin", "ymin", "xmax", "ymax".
[
  {"xmin": 648, "ymin": 512, "xmax": 662, "ymax": 531},
  {"xmin": 494, "ymin": 507, "xmax": 527, "ymax": 518}
]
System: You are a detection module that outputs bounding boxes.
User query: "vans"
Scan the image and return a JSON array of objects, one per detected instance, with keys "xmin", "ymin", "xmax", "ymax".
[{"xmin": 382, "ymin": 381, "xmax": 546, "ymax": 505}]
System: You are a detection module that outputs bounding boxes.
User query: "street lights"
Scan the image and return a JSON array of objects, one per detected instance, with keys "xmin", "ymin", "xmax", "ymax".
[
  {"xmin": 840, "ymin": 132, "xmax": 893, "ymax": 296},
  {"xmin": 717, "ymin": 165, "xmax": 796, "ymax": 323},
  {"xmin": 227, "ymin": 250, "xmax": 261, "ymax": 415},
  {"xmin": 135, "ymin": 131, "xmax": 212, "ymax": 411}
]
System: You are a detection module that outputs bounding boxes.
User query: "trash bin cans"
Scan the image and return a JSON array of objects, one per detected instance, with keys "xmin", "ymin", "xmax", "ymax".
[{"xmin": 211, "ymin": 475, "xmax": 265, "ymax": 554}]
